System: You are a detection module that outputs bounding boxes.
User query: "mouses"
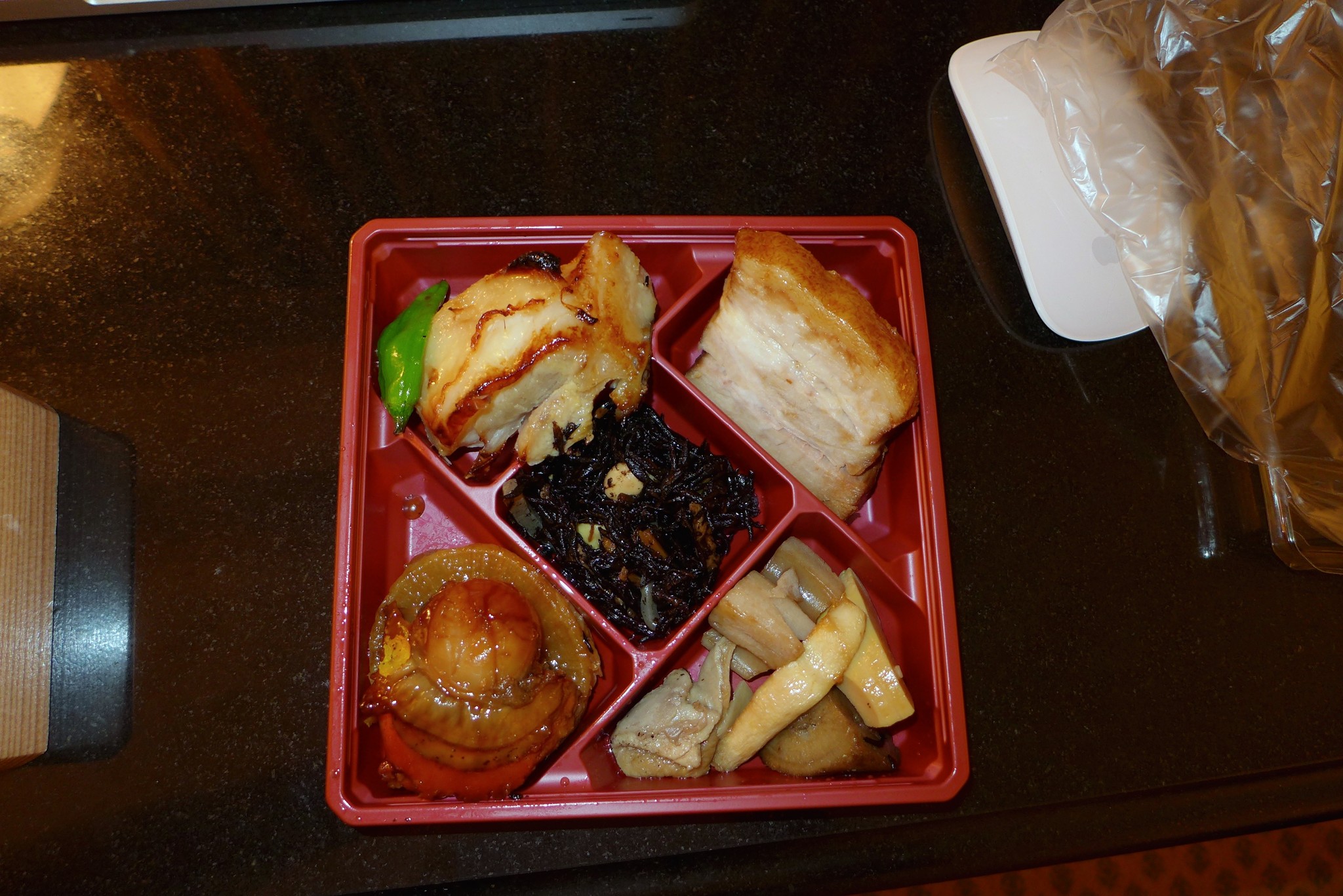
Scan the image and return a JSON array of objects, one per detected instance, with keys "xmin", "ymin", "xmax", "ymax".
[{"xmin": 945, "ymin": 32, "xmax": 1171, "ymax": 342}]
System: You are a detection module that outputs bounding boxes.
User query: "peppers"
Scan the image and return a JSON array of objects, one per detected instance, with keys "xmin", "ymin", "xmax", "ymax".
[{"xmin": 377, "ymin": 281, "xmax": 451, "ymax": 432}]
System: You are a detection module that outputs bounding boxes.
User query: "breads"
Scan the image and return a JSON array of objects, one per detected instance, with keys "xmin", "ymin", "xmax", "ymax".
[{"xmin": 683, "ymin": 224, "xmax": 920, "ymax": 525}]
